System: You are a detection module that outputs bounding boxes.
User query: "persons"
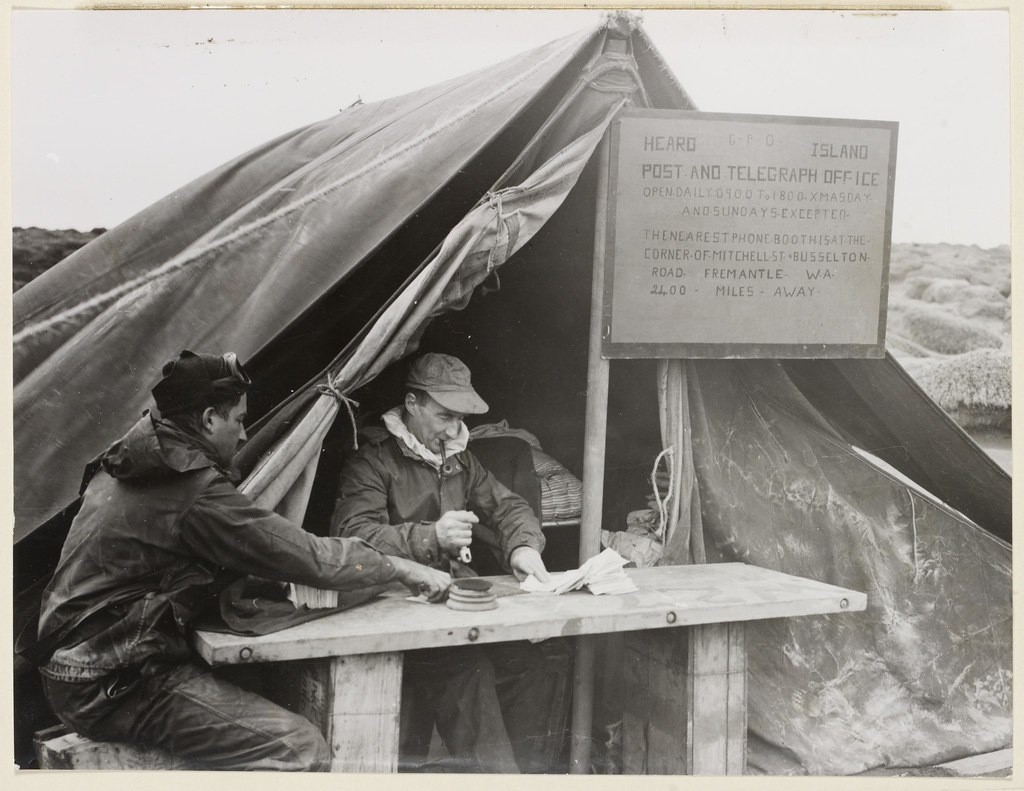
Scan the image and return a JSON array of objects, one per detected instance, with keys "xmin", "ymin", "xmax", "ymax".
[
  {"xmin": 329, "ymin": 352, "xmax": 566, "ymax": 776},
  {"xmin": 13, "ymin": 349, "xmax": 451, "ymax": 772}
]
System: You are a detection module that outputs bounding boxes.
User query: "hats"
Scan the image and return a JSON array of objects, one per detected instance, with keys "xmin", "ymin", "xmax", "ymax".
[
  {"xmin": 404, "ymin": 353, "xmax": 489, "ymax": 414},
  {"xmin": 151, "ymin": 350, "xmax": 252, "ymax": 416}
]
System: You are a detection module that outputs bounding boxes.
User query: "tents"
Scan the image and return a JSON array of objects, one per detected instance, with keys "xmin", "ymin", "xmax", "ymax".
[{"xmin": 13, "ymin": 13, "xmax": 1013, "ymax": 775}]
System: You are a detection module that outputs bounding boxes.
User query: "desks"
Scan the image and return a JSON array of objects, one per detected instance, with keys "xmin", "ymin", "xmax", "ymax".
[{"xmin": 195, "ymin": 562, "xmax": 867, "ymax": 775}]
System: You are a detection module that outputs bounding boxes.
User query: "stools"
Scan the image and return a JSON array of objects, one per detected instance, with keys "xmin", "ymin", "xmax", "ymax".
[{"xmin": 33, "ymin": 723, "xmax": 203, "ymax": 771}]
